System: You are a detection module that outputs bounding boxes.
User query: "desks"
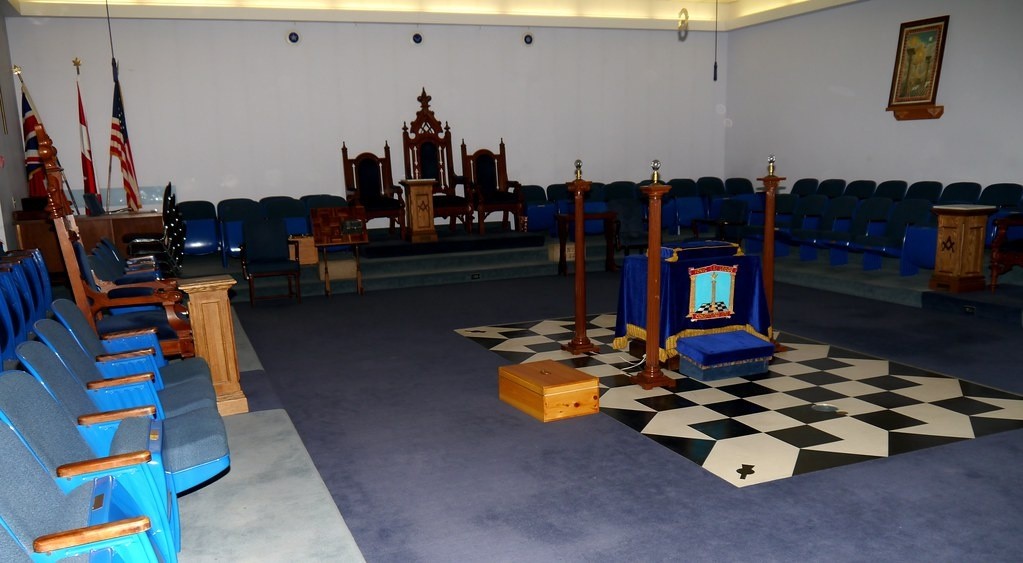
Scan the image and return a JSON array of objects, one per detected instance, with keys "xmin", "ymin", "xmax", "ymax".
[
  {"xmin": 11, "ymin": 212, "xmax": 165, "ymax": 283},
  {"xmin": 555, "ymin": 212, "xmax": 621, "ymax": 276},
  {"xmin": 315, "ymin": 242, "xmax": 369, "ymax": 296}
]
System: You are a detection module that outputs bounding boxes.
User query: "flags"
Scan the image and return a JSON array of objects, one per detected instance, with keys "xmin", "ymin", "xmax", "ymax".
[
  {"xmin": 78, "ymin": 87, "xmax": 102, "ymax": 215},
  {"xmin": 22, "ymin": 92, "xmax": 46, "ymax": 197},
  {"xmin": 110, "ymin": 82, "xmax": 142, "ymax": 212}
]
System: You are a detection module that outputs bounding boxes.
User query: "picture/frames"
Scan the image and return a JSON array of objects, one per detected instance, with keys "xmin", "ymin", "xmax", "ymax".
[{"xmin": 888, "ymin": 15, "xmax": 950, "ymax": 107}]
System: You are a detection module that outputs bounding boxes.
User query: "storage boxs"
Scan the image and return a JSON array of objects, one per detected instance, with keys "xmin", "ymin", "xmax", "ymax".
[
  {"xmin": 497, "ymin": 359, "xmax": 599, "ymax": 422},
  {"xmin": 13, "ymin": 210, "xmax": 48, "ymax": 221},
  {"xmin": 18, "ymin": 194, "xmax": 48, "ymax": 211},
  {"xmin": 310, "ymin": 206, "xmax": 367, "ymax": 243}
]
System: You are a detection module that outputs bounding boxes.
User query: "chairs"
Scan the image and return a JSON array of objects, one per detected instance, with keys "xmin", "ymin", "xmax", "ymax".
[
  {"xmin": 550, "ymin": 182, "xmax": 575, "ymax": 240},
  {"xmin": 610, "ymin": 181, "xmax": 644, "ymax": 235},
  {"xmin": 239, "ymin": 212, "xmax": 303, "ymax": 307},
  {"xmin": 219, "ymin": 198, "xmax": 259, "ymax": 269},
  {"xmin": 260, "ymin": 194, "xmax": 355, "ymax": 250},
  {"xmin": 0, "ymin": 178, "xmax": 233, "ymax": 563},
  {"xmin": 341, "ymin": 141, "xmax": 407, "ymax": 240},
  {"xmin": 401, "ymin": 86, "xmax": 476, "ymax": 234},
  {"xmin": 671, "ymin": 179, "xmax": 1023, "ymax": 295},
  {"xmin": 522, "ymin": 185, "xmax": 555, "ymax": 234},
  {"xmin": 584, "ymin": 183, "xmax": 608, "ymax": 235},
  {"xmin": 638, "ymin": 180, "xmax": 677, "ymax": 236},
  {"xmin": 461, "ymin": 138, "xmax": 529, "ymax": 233}
]
